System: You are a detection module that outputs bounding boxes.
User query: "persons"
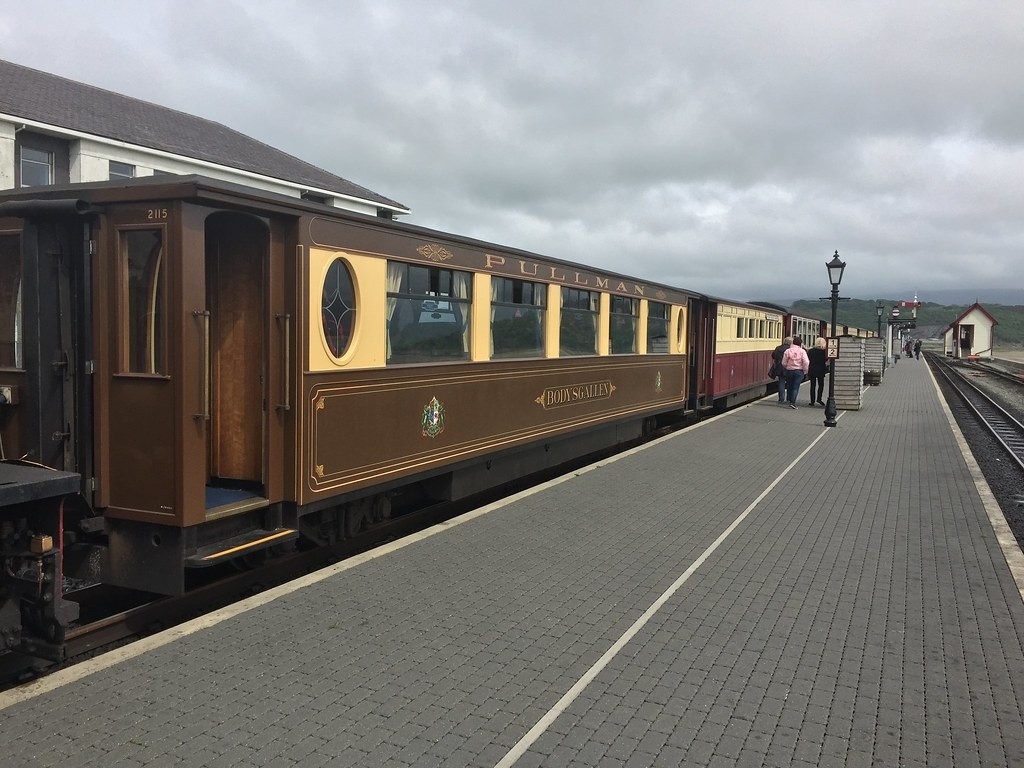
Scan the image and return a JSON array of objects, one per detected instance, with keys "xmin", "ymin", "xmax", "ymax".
[
  {"xmin": 772, "ymin": 337, "xmax": 793, "ymax": 404},
  {"xmin": 782, "ymin": 337, "xmax": 810, "ymax": 409},
  {"xmin": 807, "ymin": 338, "xmax": 828, "ymax": 407},
  {"xmin": 904, "ymin": 337, "xmax": 922, "ymax": 360}
]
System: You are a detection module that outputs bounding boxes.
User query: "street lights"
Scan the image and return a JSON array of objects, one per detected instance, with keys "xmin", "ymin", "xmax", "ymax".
[
  {"xmin": 876, "ymin": 301, "xmax": 884, "ymax": 338},
  {"xmin": 818, "ymin": 249, "xmax": 850, "ymax": 428}
]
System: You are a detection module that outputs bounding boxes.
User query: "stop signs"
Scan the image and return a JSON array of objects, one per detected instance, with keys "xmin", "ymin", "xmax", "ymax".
[{"xmin": 892, "ymin": 310, "xmax": 900, "ymax": 317}]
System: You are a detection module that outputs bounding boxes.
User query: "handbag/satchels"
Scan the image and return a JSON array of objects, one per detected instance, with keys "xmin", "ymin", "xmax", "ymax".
[{"xmin": 768, "ymin": 363, "xmax": 776, "ymax": 380}]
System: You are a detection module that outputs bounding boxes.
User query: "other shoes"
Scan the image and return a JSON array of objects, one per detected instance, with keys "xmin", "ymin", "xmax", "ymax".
[
  {"xmin": 809, "ymin": 403, "xmax": 815, "ymax": 406},
  {"xmin": 817, "ymin": 400, "xmax": 825, "ymax": 406},
  {"xmin": 778, "ymin": 400, "xmax": 785, "ymax": 404},
  {"xmin": 789, "ymin": 403, "xmax": 799, "ymax": 410}
]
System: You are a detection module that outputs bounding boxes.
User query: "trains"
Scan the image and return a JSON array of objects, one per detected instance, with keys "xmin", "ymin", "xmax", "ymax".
[{"xmin": 0, "ymin": 173, "xmax": 879, "ymax": 679}]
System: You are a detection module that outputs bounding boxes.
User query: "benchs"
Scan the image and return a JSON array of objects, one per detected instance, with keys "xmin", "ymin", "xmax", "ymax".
[{"xmin": 389, "ymin": 321, "xmax": 465, "ymax": 363}]
